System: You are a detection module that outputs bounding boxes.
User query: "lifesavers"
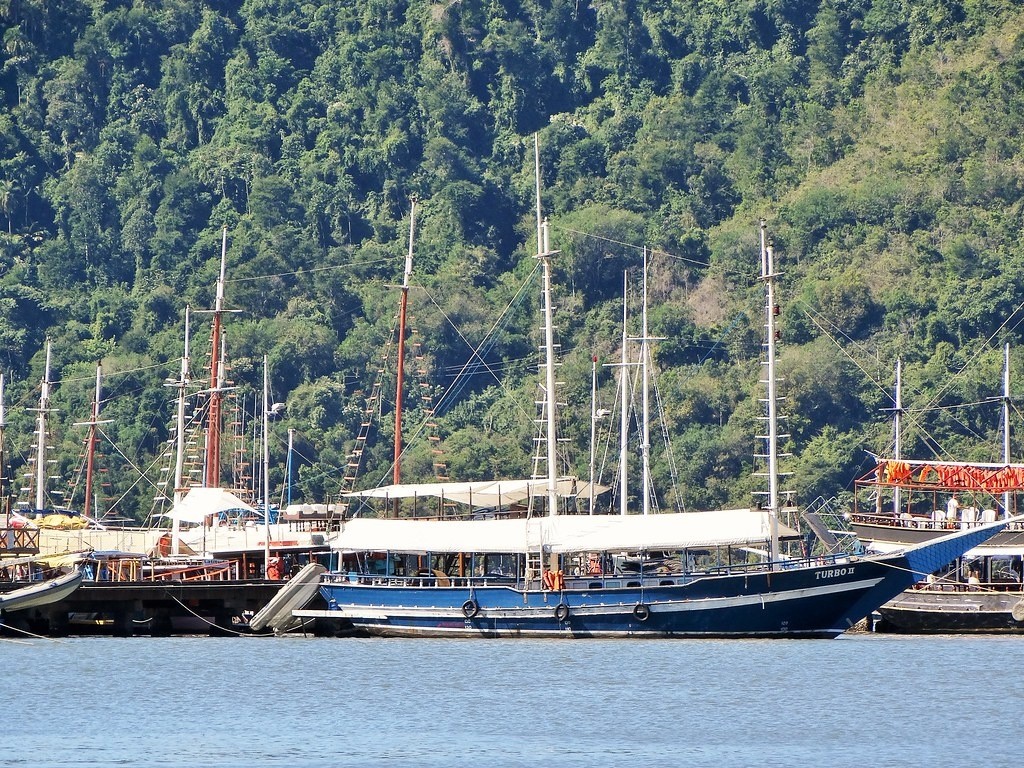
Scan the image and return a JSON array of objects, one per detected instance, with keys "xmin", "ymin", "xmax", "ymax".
[
  {"xmin": 555, "ymin": 603, "xmax": 568, "ymax": 621},
  {"xmin": 461, "ymin": 600, "xmax": 477, "ymax": 617},
  {"xmin": 633, "ymin": 604, "xmax": 650, "ymax": 621}
]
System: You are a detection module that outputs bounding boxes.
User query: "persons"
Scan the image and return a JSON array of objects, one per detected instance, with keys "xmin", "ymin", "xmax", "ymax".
[
  {"xmin": 267, "ymin": 557, "xmax": 281, "ymax": 580},
  {"xmin": 966, "ymin": 564, "xmax": 974, "ymax": 591},
  {"xmin": 1011, "ymin": 554, "xmax": 1024, "ymax": 591},
  {"xmin": 947, "ymin": 496, "xmax": 963, "ymax": 528},
  {"xmin": 927, "ymin": 572, "xmax": 935, "ymax": 590}
]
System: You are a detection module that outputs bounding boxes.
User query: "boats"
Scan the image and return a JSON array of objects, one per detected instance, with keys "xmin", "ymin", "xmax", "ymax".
[
  {"xmin": 851, "ymin": 343, "xmax": 1024, "ymax": 634},
  {"xmin": 248, "ymin": 563, "xmax": 326, "ymax": 634}
]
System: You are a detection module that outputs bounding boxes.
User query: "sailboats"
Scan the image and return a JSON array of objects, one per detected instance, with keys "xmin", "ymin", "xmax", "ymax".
[
  {"xmin": 0, "ymin": 221, "xmax": 695, "ymax": 614},
  {"xmin": 315, "ymin": 129, "xmax": 1024, "ymax": 638}
]
